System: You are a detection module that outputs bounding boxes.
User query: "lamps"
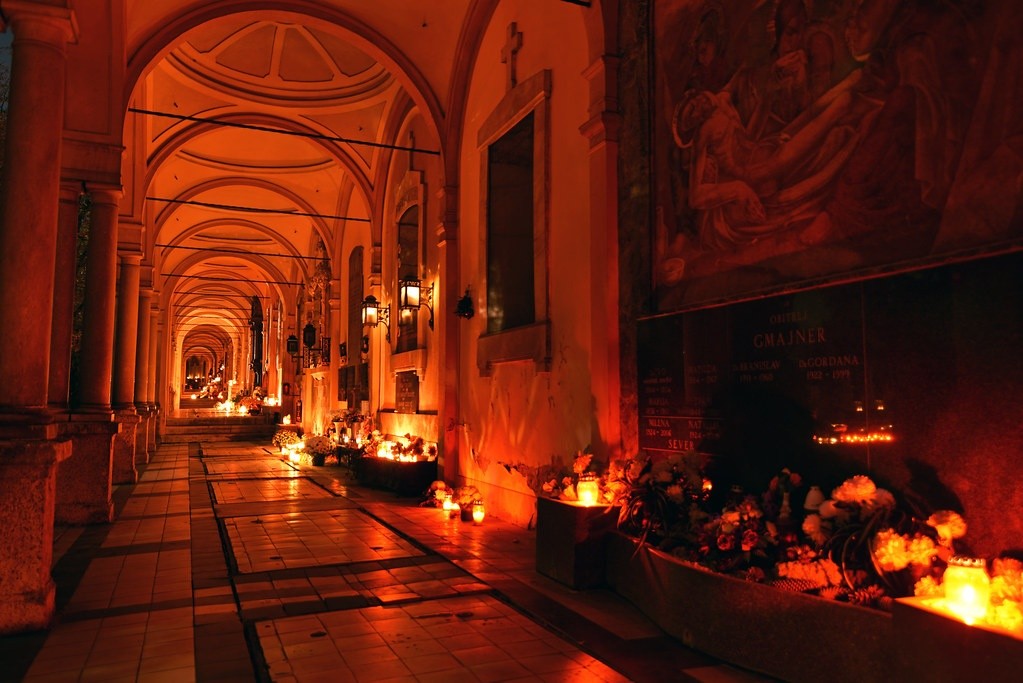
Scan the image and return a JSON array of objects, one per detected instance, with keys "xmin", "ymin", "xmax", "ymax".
[
  {"xmin": 399, "ymin": 271, "xmax": 435, "ymax": 331},
  {"xmin": 287, "ymin": 333, "xmax": 310, "ymax": 368},
  {"xmin": 359, "ymin": 295, "xmax": 392, "ymax": 344},
  {"xmin": 302, "ymin": 322, "xmax": 329, "ymax": 363}
]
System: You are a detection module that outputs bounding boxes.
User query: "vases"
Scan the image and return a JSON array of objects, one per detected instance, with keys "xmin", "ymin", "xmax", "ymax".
[
  {"xmin": 310, "ymin": 451, "xmax": 326, "ymax": 466},
  {"xmin": 249, "ymin": 409, "xmax": 260, "ymax": 416},
  {"xmin": 604, "ymin": 530, "xmax": 896, "ymax": 683},
  {"xmin": 459, "ymin": 502, "xmax": 475, "ymax": 522},
  {"xmin": 279, "ymin": 445, "xmax": 284, "ymax": 451},
  {"xmin": 433, "ymin": 499, "xmax": 443, "ymax": 508}
]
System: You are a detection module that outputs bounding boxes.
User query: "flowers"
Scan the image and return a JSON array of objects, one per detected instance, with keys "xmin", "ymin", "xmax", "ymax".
[
  {"xmin": 272, "ymin": 428, "xmax": 300, "ymax": 448},
  {"xmin": 591, "ymin": 444, "xmax": 969, "ymax": 608},
  {"xmin": 451, "ymin": 484, "xmax": 484, "ymax": 506},
  {"xmin": 301, "ymin": 435, "xmax": 338, "ymax": 456},
  {"xmin": 241, "ymin": 398, "xmax": 267, "ymax": 414},
  {"xmin": 425, "ymin": 480, "xmax": 454, "ymax": 504}
]
